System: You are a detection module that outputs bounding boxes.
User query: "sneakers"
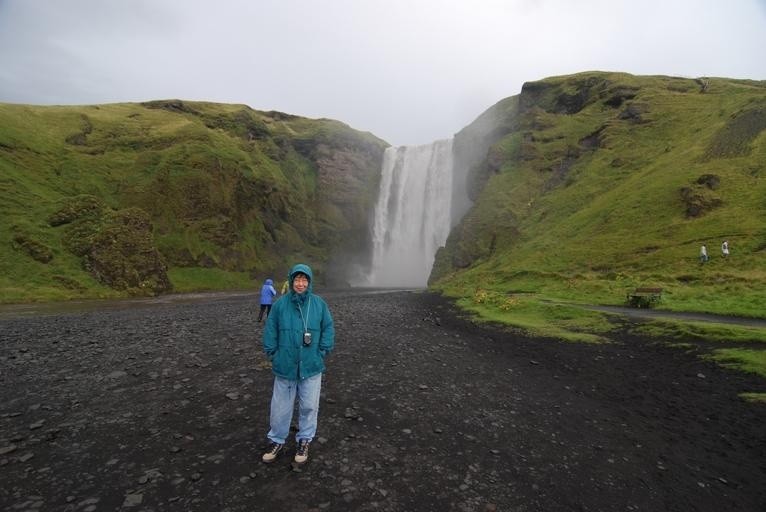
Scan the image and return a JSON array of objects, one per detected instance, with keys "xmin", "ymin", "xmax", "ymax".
[
  {"xmin": 294, "ymin": 438, "xmax": 309, "ymax": 464},
  {"xmin": 262, "ymin": 442, "xmax": 282, "ymax": 463}
]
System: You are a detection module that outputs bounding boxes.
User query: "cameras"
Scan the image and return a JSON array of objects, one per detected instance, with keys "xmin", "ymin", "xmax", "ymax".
[{"xmin": 304, "ymin": 332, "xmax": 312, "ymax": 345}]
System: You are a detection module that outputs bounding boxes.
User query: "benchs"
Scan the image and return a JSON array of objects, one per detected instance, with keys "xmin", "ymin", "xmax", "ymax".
[{"xmin": 628, "ymin": 287, "xmax": 662, "ymax": 308}]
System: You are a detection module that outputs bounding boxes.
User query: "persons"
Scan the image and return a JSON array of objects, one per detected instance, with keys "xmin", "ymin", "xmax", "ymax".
[
  {"xmin": 281, "ymin": 280, "xmax": 289, "ymax": 295},
  {"xmin": 262, "ymin": 264, "xmax": 336, "ymax": 465},
  {"xmin": 722, "ymin": 240, "xmax": 730, "ymax": 257},
  {"xmin": 701, "ymin": 245, "xmax": 708, "ymax": 264},
  {"xmin": 257, "ymin": 279, "xmax": 276, "ymax": 321}
]
